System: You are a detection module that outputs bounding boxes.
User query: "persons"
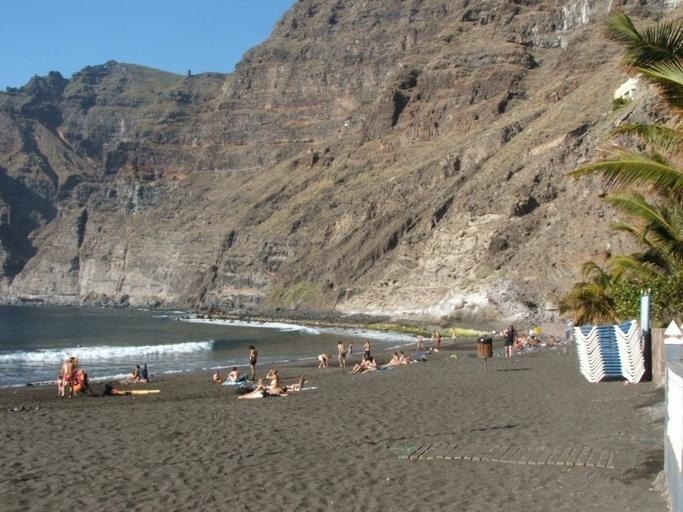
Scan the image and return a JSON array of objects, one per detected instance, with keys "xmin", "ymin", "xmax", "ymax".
[
  {"xmin": 316, "ymin": 325, "xmax": 457, "ymax": 376},
  {"xmin": 248, "ymin": 344, "xmax": 259, "ymax": 381},
  {"xmin": 57, "ymin": 356, "xmax": 149, "ymax": 397},
  {"xmin": 210, "ymin": 366, "xmax": 307, "ymax": 400},
  {"xmin": 500, "ymin": 322, "xmax": 564, "ymax": 358}
]
